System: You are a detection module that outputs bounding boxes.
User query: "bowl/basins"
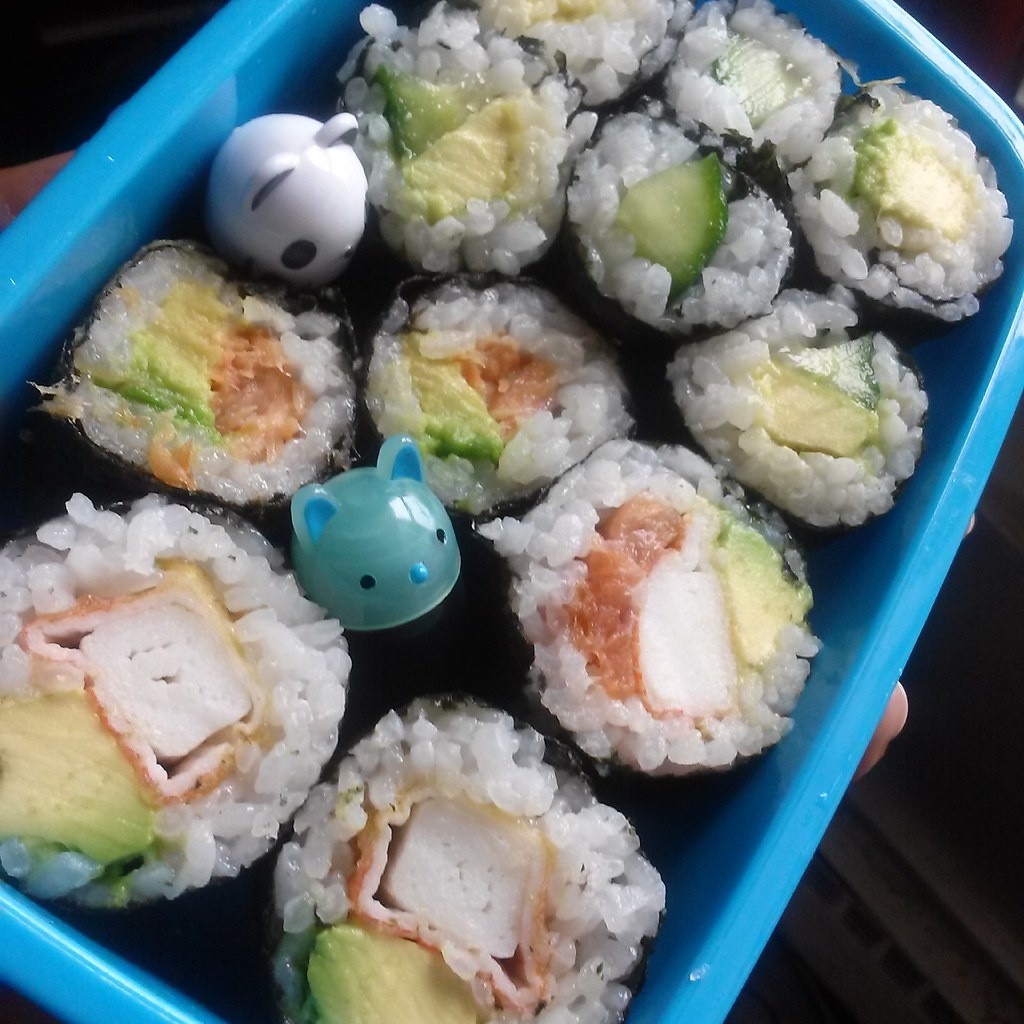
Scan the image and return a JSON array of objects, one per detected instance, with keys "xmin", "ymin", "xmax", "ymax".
[{"xmin": 1, "ymin": 1, "xmax": 1024, "ymax": 1023}]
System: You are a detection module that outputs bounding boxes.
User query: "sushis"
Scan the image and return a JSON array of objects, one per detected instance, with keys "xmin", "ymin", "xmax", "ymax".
[{"xmin": 0, "ymin": 0, "xmax": 1015, "ymax": 1024}]
